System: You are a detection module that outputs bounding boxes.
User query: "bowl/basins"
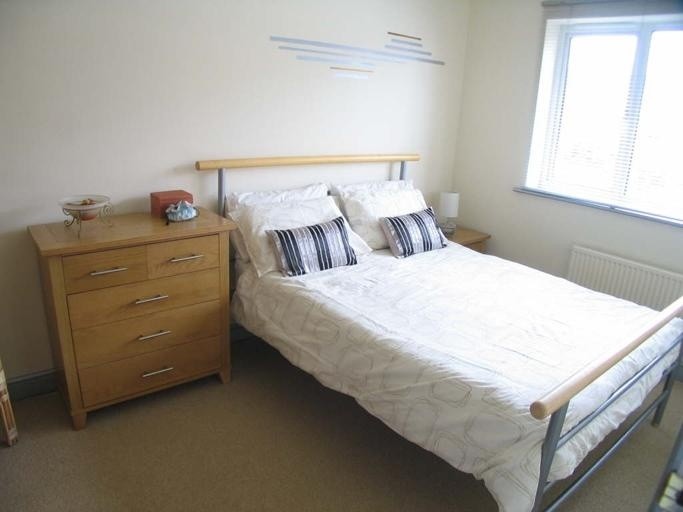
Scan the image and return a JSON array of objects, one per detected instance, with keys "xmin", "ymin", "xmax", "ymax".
[{"xmin": 57, "ymin": 194, "xmax": 111, "ymax": 221}]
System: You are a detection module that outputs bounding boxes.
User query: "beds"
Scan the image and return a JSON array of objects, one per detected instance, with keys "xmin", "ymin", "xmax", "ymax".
[{"xmin": 195, "ymin": 152, "xmax": 683, "ymax": 511}]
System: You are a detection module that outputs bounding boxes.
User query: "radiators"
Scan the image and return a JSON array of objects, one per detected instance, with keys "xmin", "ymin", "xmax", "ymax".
[{"xmin": 566, "ymin": 243, "xmax": 683, "ymax": 313}]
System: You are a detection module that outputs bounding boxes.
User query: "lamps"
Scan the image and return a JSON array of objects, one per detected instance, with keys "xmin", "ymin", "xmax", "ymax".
[{"xmin": 437, "ymin": 191, "xmax": 460, "ymax": 236}]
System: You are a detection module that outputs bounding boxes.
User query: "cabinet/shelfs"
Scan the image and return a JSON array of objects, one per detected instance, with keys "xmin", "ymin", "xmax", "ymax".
[{"xmin": 26, "ymin": 204, "xmax": 238, "ymax": 433}]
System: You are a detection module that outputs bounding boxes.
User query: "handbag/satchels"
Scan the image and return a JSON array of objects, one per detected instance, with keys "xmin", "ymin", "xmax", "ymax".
[{"xmin": 165, "ymin": 200, "xmax": 196, "ymax": 221}]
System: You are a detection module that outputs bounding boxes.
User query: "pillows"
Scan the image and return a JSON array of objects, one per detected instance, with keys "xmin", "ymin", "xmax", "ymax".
[{"xmin": 224, "ymin": 179, "xmax": 448, "ymax": 279}]
